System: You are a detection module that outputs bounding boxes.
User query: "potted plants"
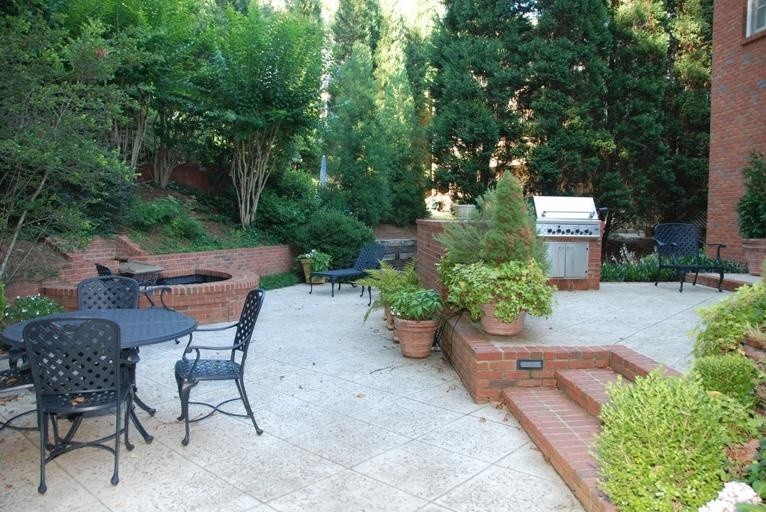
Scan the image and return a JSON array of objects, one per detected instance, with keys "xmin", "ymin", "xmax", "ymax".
[
  {"xmin": 352, "ymin": 166, "xmax": 558, "ymax": 360},
  {"xmin": 733, "ymin": 142, "xmax": 766, "ymax": 276},
  {"xmin": 295, "ymin": 249, "xmax": 333, "ymax": 285}
]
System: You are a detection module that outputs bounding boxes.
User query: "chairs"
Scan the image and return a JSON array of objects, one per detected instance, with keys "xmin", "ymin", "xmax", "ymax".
[
  {"xmin": 171, "ymin": 287, "xmax": 266, "ymax": 447},
  {"xmin": 307, "ymin": 239, "xmax": 386, "ymax": 298},
  {"xmin": 95, "ymin": 261, "xmax": 180, "ymax": 347},
  {"xmin": 22, "ymin": 316, "xmax": 136, "ymax": 496},
  {"xmin": 653, "ymin": 222, "xmax": 727, "ymax": 294},
  {"xmin": 75, "ymin": 275, "xmax": 139, "ymax": 383}
]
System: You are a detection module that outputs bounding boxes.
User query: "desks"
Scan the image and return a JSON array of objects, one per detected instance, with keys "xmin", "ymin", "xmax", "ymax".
[{"xmin": 0, "ymin": 305, "xmax": 199, "ymax": 444}]
